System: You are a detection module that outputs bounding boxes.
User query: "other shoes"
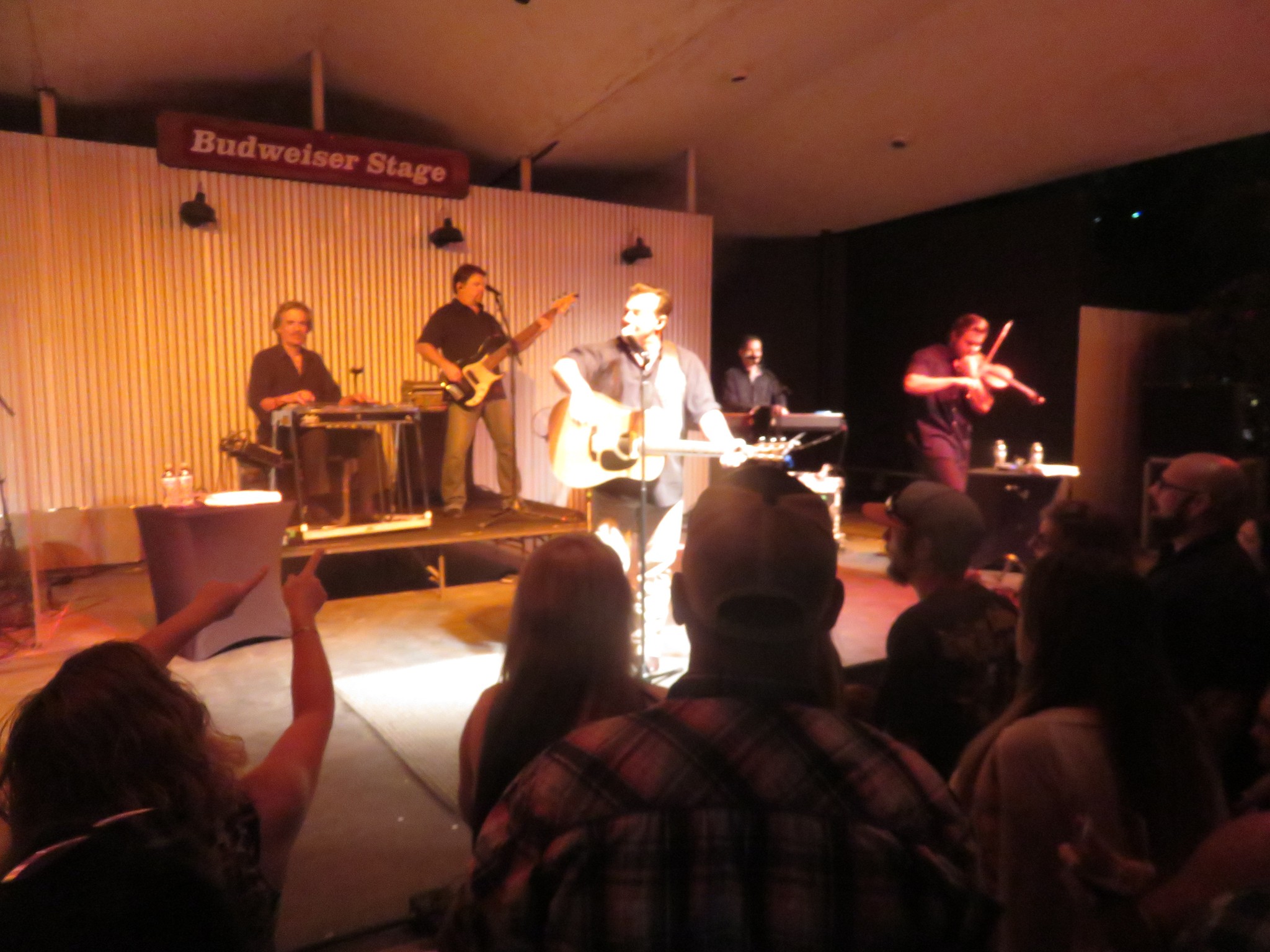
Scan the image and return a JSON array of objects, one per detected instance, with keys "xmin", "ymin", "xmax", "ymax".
[
  {"xmin": 447, "ymin": 502, "xmax": 468, "ymax": 525},
  {"xmin": 354, "ymin": 506, "xmax": 389, "ymax": 523},
  {"xmin": 304, "ymin": 505, "xmax": 332, "ymax": 525},
  {"xmin": 505, "ymin": 500, "xmax": 536, "ymax": 521}
]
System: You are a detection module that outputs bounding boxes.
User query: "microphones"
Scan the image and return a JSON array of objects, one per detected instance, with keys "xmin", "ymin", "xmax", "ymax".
[
  {"xmin": 621, "ymin": 325, "xmax": 649, "ymax": 361},
  {"xmin": 485, "ymin": 284, "xmax": 501, "ymax": 296}
]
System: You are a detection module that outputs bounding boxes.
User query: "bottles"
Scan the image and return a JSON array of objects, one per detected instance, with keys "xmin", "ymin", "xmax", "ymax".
[
  {"xmin": 993, "ymin": 439, "xmax": 1007, "ymax": 469},
  {"xmin": 160, "ymin": 463, "xmax": 183, "ymax": 507},
  {"xmin": 177, "ymin": 463, "xmax": 194, "ymax": 506},
  {"xmin": 1029, "ymin": 441, "xmax": 1044, "ymax": 465}
]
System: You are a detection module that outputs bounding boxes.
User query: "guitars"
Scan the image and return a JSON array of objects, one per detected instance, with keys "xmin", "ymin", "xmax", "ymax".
[
  {"xmin": 548, "ymin": 390, "xmax": 796, "ymax": 489},
  {"xmin": 439, "ymin": 292, "xmax": 580, "ymax": 407}
]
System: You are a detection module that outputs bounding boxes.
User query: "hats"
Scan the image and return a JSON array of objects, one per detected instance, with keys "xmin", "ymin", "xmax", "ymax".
[{"xmin": 884, "ymin": 478, "xmax": 989, "ymax": 546}]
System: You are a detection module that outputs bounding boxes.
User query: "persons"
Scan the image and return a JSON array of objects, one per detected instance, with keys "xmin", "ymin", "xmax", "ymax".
[
  {"xmin": 723, "ymin": 335, "xmax": 788, "ymax": 415},
  {"xmin": 903, "ymin": 314, "xmax": 994, "ymax": 492},
  {"xmin": 247, "ymin": 302, "xmax": 390, "ymax": 525},
  {"xmin": 0, "ymin": 545, "xmax": 334, "ymax": 952},
  {"xmin": 453, "ymin": 455, "xmax": 1270, "ymax": 952},
  {"xmin": 554, "ymin": 283, "xmax": 747, "ymax": 666},
  {"xmin": 413, "ymin": 263, "xmax": 551, "ymax": 515}
]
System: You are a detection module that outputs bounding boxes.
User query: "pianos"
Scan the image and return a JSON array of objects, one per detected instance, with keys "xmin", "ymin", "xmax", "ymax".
[
  {"xmin": 269, "ymin": 401, "xmax": 432, "ymax": 547},
  {"xmin": 687, "ymin": 411, "xmax": 848, "ymax": 475}
]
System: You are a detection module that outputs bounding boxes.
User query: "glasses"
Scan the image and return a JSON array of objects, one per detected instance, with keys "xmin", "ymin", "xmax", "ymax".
[{"xmin": 1153, "ymin": 476, "xmax": 1193, "ymax": 498}]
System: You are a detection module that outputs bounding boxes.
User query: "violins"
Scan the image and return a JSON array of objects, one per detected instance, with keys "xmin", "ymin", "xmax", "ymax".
[{"xmin": 953, "ymin": 352, "xmax": 1046, "ymax": 405}]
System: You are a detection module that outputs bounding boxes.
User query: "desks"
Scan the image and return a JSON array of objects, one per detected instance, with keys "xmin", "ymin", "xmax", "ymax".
[
  {"xmin": 967, "ymin": 464, "xmax": 1080, "ymax": 578},
  {"xmin": 134, "ymin": 499, "xmax": 296, "ymax": 662}
]
